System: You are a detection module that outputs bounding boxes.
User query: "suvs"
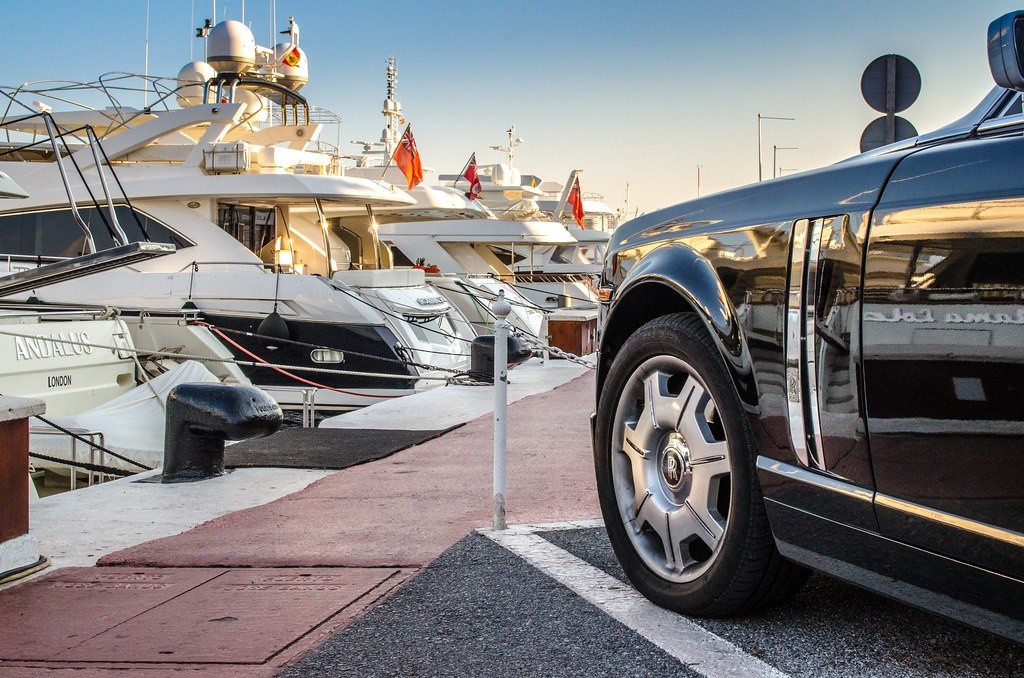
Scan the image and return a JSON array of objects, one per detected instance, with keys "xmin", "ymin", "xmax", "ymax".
[{"xmin": 589, "ymin": 6, "xmax": 1024, "ymax": 643}]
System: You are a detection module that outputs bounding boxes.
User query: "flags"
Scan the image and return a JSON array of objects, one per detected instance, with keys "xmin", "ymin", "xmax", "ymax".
[
  {"xmin": 566, "ymin": 175, "xmax": 586, "ymax": 229},
  {"xmin": 391, "ymin": 126, "xmax": 424, "ymax": 190},
  {"xmin": 462, "ymin": 155, "xmax": 481, "ymax": 198}
]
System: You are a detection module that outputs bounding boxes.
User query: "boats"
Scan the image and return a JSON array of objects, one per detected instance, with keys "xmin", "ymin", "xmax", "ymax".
[{"xmin": 0, "ymin": 5, "xmax": 624, "ymax": 483}]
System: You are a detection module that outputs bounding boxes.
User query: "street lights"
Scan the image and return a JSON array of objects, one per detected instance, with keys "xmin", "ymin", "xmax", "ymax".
[
  {"xmin": 696, "ymin": 163, "xmax": 704, "ymax": 197},
  {"xmin": 773, "ymin": 145, "xmax": 798, "ymax": 178},
  {"xmin": 757, "ymin": 112, "xmax": 796, "ymax": 182},
  {"xmin": 779, "ymin": 166, "xmax": 799, "ymax": 178}
]
